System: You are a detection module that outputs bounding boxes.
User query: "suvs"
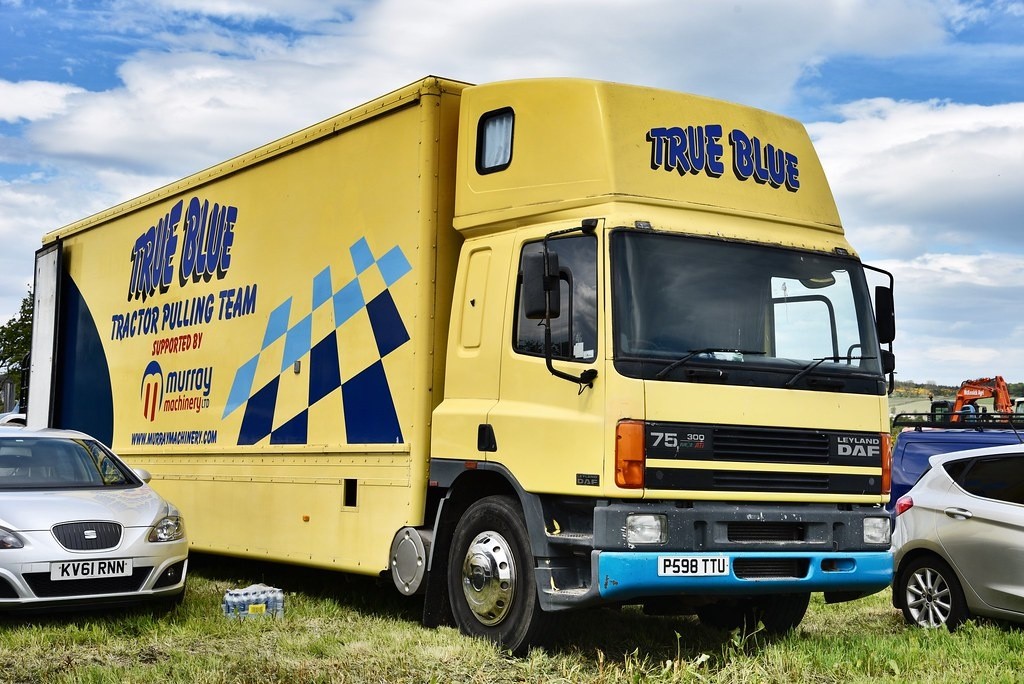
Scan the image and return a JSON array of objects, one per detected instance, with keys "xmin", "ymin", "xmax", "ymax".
[{"xmin": 889, "ymin": 413, "xmax": 1024, "ymax": 534}]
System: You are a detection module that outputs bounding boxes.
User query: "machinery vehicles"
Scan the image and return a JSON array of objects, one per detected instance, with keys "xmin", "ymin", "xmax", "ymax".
[{"xmin": 931, "ymin": 377, "xmax": 1024, "ymax": 422}]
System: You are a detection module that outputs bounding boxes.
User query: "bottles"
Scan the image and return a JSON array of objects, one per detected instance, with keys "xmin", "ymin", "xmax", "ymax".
[{"xmin": 223, "ymin": 582, "xmax": 285, "ymax": 621}]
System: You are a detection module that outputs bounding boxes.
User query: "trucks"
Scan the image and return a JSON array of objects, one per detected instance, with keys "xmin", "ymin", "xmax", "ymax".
[{"xmin": 27, "ymin": 77, "xmax": 897, "ymax": 661}]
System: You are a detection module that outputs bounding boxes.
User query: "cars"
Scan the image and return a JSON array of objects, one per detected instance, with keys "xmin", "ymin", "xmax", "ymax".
[
  {"xmin": 1, "ymin": 413, "xmax": 189, "ymax": 617},
  {"xmin": 893, "ymin": 442, "xmax": 1024, "ymax": 634}
]
